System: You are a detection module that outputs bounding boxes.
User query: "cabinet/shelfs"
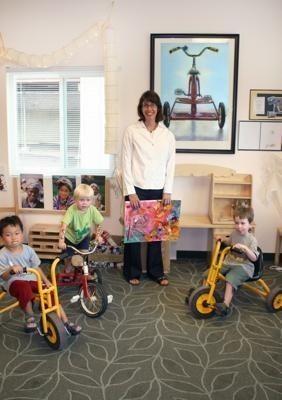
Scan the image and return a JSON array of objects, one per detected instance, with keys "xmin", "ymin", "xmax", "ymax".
[
  {"xmin": 28, "ymin": 223, "xmax": 66, "ymax": 261},
  {"xmin": 209, "ymin": 174, "xmax": 254, "ymax": 225}
]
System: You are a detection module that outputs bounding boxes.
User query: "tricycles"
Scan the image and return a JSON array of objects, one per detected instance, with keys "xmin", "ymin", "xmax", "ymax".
[
  {"xmin": 162, "ymin": 45, "xmax": 226, "ymax": 129},
  {"xmin": 187, "ymin": 235, "xmax": 281, "ymax": 317},
  {"xmin": 50, "ymin": 234, "xmax": 108, "ymax": 318},
  {"xmin": 1, "ymin": 252, "xmax": 71, "ymax": 349}
]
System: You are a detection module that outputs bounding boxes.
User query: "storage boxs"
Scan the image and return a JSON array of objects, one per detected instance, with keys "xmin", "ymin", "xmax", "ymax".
[{"xmin": 87, "ymin": 234, "xmax": 126, "ymax": 263}]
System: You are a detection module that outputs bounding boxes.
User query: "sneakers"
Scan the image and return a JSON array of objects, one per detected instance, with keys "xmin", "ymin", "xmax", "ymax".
[{"xmin": 215, "ymin": 303, "xmax": 231, "ymax": 315}]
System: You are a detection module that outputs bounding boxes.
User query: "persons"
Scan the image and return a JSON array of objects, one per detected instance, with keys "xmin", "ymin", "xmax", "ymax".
[
  {"xmin": 199, "ymin": 206, "xmax": 260, "ymax": 316},
  {"xmin": 53, "ymin": 178, "xmax": 74, "ymax": 210},
  {"xmin": 58, "ymin": 184, "xmax": 104, "ymax": 283},
  {"xmin": 21, "ymin": 177, "xmax": 43, "ymax": 208},
  {"xmin": 90, "ymin": 183, "xmax": 102, "ymax": 212},
  {"xmin": 0, "ymin": 215, "xmax": 82, "ymax": 337},
  {"xmin": 122, "ymin": 91, "xmax": 176, "ymax": 287}
]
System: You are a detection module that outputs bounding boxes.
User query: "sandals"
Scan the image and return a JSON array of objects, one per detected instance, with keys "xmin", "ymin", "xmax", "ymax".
[
  {"xmin": 156, "ymin": 275, "xmax": 170, "ymax": 285},
  {"xmin": 63, "ymin": 319, "xmax": 82, "ymax": 334},
  {"xmin": 25, "ymin": 312, "xmax": 38, "ymax": 330}
]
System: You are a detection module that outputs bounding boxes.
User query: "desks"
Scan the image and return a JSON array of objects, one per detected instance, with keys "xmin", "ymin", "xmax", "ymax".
[{"xmin": 139, "ymin": 215, "xmax": 255, "ymax": 276}]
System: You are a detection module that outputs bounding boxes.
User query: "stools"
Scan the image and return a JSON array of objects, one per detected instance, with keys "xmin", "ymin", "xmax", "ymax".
[{"xmin": 274, "ymin": 228, "xmax": 282, "ymax": 262}]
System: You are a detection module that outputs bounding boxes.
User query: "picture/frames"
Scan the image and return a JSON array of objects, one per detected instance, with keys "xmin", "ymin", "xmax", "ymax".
[
  {"xmin": 149, "ymin": 33, "xmax": 241, "ymax": 154},
  {"xmin": 248, "ymin": 89, "xmax": 282, "ymax": 120}
]
